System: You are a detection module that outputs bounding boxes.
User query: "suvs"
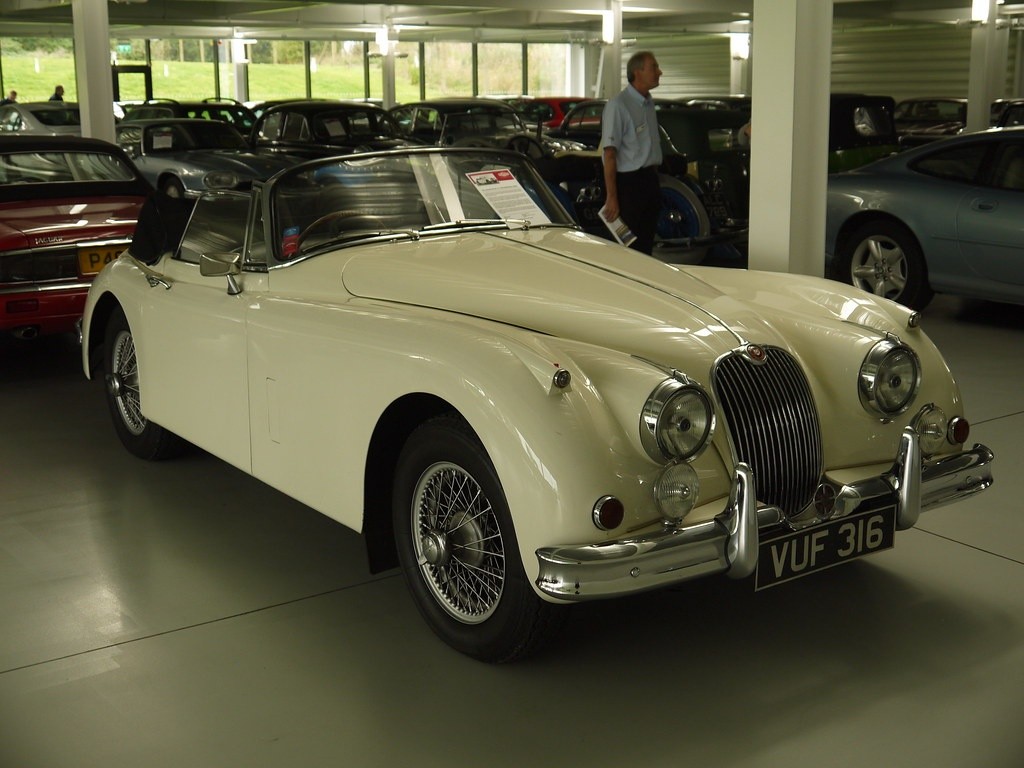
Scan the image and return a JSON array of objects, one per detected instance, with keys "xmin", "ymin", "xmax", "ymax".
[{"xmin": 111, "ymin": 96, "xmax": 260, "ymax": 148}]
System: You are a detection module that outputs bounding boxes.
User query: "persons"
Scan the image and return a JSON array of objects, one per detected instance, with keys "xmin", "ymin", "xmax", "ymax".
[
  {"xmin": 738, "ymin": 121, "xmax": 751, "ymax": 145},
  {"xmin": 601, "ymin": 52, "xmax": 663, "ymax": 254},
  {"xmin": 0, "ymin": 91, "xmax": 17, "ymax": 105},
  {"xmin": 49, "ymin": 87, "xmax": 67, "ymax": 119}
]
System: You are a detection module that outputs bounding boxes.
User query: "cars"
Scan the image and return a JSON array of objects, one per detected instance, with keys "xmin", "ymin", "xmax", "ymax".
[
  {"xmin": 0, "ymin": 131, "xmax": 156, "ymax": 341},
  {"xmin": 114, "ymin": 120, "xmax": 321, "ymax": 204},
  {"xmin": 244, "ymin": 83, "xmax": 1023, "ymax": 264},
  {"xmin": 824, "ymin": 124, "xmax": 1024, "ymax": 319},
  {"xmin": 0, "ymin": 101, "xmax": 122, "ymax": 183},
  {"xmin": 81, "ymin": 145, "xmax": 995, "ymax": 668}
]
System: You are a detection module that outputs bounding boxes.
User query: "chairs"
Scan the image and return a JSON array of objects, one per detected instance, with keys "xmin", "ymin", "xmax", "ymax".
[{"xmin": 916, "ymin": 102, "xmax": 940, "ymax": 117}]
